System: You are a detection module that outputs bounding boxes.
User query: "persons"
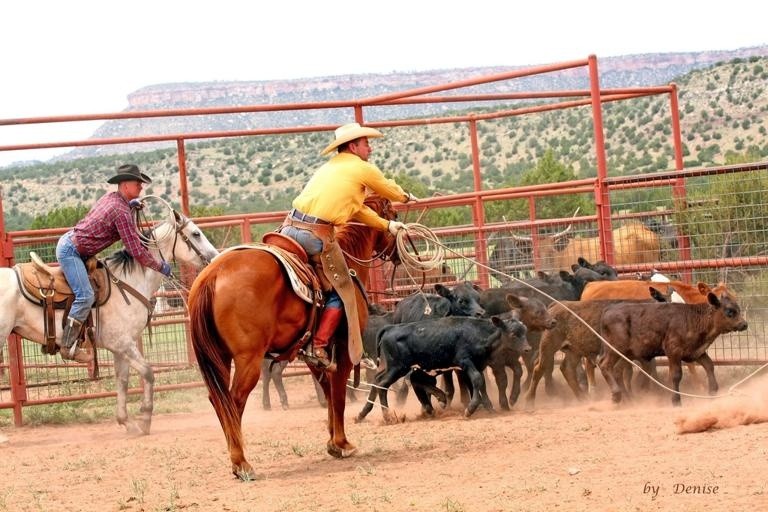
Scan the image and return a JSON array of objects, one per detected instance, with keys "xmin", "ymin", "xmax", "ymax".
[
  {"xmin": 54, "ymin": 163, "xmax": 173, "ymax": 363},
  {"xmin": 280, "ymin": 121, "xmax": 422, "ymax": 375}
]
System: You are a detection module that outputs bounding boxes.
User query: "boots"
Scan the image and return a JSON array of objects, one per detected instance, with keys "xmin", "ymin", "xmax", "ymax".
[
  {"xmin": 305, "ymin": 308, "xmax": 343, "ymax": 372},
  {"xmin": 60, "ymin": 316, "xmax": 95, "ymax": 363}
]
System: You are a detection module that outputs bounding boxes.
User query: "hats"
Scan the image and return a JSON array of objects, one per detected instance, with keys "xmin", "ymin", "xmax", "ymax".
[
  {"xmin": 321, "ymin": 123, "xmax": 383, "ymax": 156},
  {"xmin": 108, "ymin": 165, "xmax": 152, "ymax": 184}
]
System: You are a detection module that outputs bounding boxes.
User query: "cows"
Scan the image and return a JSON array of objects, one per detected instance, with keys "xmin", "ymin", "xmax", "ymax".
[{"xmin": 352, "ymin": 205, "xmax": 748, "ymax": 425}]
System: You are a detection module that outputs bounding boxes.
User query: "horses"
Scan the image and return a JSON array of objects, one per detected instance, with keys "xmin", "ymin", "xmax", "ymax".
[
  {"xmin": 187, "ymin": 190, "xmax": 411, "ymax": 481},
  {"xmin": 0, "ymin": 209, "xmax": 220, "ymax": 438}
]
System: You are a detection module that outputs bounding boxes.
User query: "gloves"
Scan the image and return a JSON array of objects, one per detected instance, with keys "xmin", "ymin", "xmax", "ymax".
[
  {"xmin": 388, "ymin": 220, "xmax": 408, "ymax": 238},
  {"xmin": 408, "ymin": 193, "xmax": 417, "ymax": 201},
  {"xmin": 129, "ymin": 199, "xmax": 143, "ymax": 210},
  {"xmin": 158, "ymin": 262, "xmax": 171, "ymax": 277}
]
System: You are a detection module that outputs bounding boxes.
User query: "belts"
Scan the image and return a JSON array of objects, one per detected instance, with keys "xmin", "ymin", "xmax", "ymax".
[
  {"xmin": 68, "ymin": 231, "xmax": 84, "ymax": 255},
  {"xmin": 293, "ymin": 210, "xmax": 328, "ymax": 225}
]
